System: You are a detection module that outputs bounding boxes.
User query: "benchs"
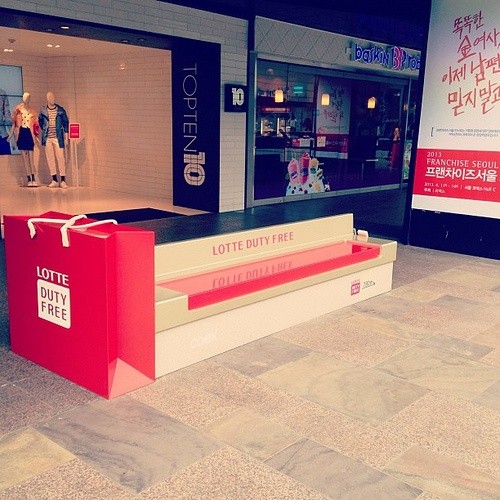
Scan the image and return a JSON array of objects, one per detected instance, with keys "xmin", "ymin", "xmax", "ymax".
[{"xmin": 154, "ymin": 213, "xmax": 397, "ymax": 378}]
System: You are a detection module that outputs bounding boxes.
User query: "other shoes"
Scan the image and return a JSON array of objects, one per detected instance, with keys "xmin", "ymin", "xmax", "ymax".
[
  {"xmin": 32, "ymin": 181, "xmax": 38, "ymax": 186},
  {"xmin": 48, "ymin": 180, "xmax": 59, "ymax": 188},
  {"xmin": 28, "ymin": 180, "xmax": 32, "ymax": 186},
  {"xmin": 59, "ymin": 181, "xmax": 68, "ymax": 188}
]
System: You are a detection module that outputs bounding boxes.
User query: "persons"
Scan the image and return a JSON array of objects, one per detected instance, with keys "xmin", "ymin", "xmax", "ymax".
[
  {"xmin": 1, "ymin": 88, "xmax": 13, "ymax": 154},
  {"xmin": 38, "ymin": 91, "xmax": 69, "ymax": 188},
  {"xmin": 256, "ymin": 114, "xmax": 313, "ymax": 137},
  {"xmin": 7, "ymin": 93, "xmax": 41, "ymax": 186}
]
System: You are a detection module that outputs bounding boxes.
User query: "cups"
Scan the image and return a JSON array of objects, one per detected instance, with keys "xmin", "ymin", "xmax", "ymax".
[
  {"xmin": 288, "ymin": 162, "xmax": 299, "ymax": 184},
  {"xmin": 300, "ymin": 158, "xmax": 311, "ymax": 185},
  {"xmin": 309, "ymin": 161, "xmax": 318, "ymax": 183}
]
